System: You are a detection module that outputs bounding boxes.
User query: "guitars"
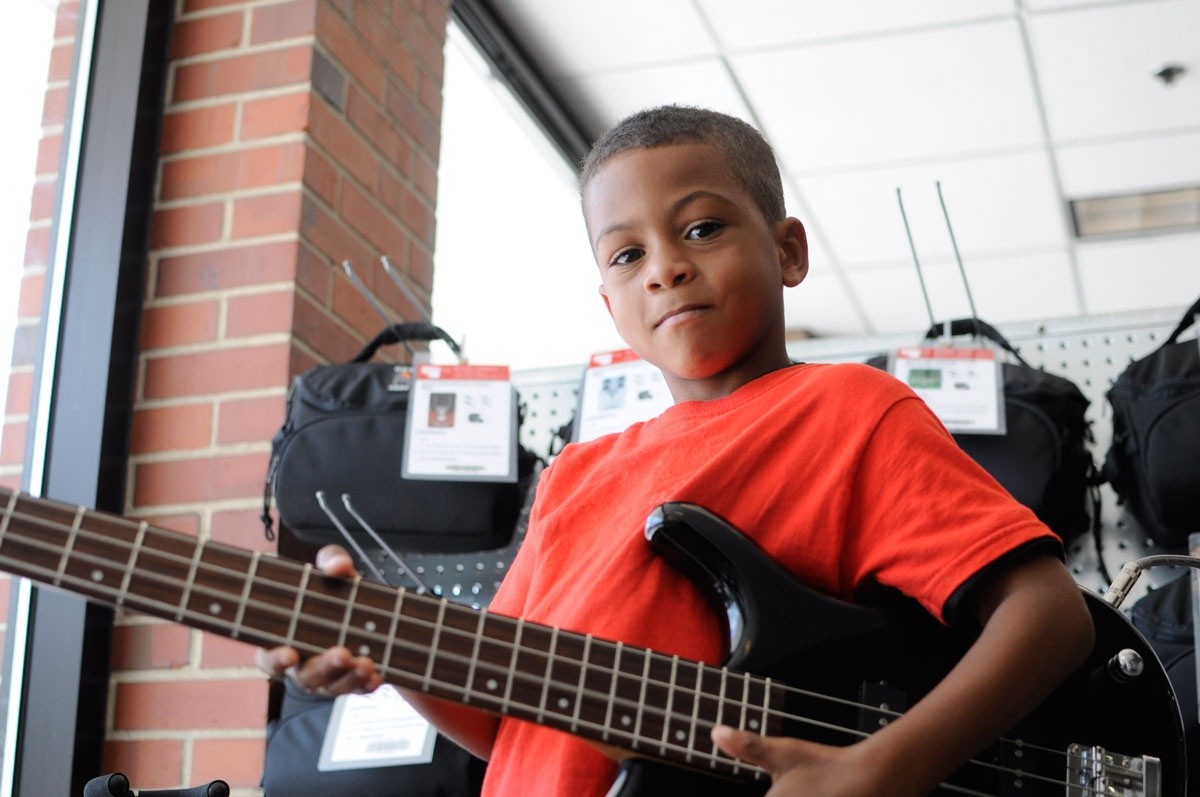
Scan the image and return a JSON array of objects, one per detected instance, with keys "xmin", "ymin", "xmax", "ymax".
[{"xmin": 0, "ymin": 483, "xmax": 1191, "ymax": 797}]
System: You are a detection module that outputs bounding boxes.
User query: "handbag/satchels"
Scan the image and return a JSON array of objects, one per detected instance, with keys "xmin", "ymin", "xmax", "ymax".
[
  {"xmin": 865, "ymin": 320, "xmax": 1098, "ymax": 551},
  {"xmin": 1131, "ymin": 569, "xmax": 1200, "ymax": 766},
  {"xmin": 263, "ymin": 320, "xmax": 539, "ymax": 553},
  {"xmin": 1099, "ymin": 298, "xmax": 1200, "ymax": 554},
  {"xmin": 264, "ymin": 680, "xmax": 482, "ymax": 797}
]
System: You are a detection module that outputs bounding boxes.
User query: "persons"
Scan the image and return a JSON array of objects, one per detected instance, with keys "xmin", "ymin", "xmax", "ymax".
[{"xmin": 254, "ymin": 106, "xmax": 1095, "ymax": 796}]
